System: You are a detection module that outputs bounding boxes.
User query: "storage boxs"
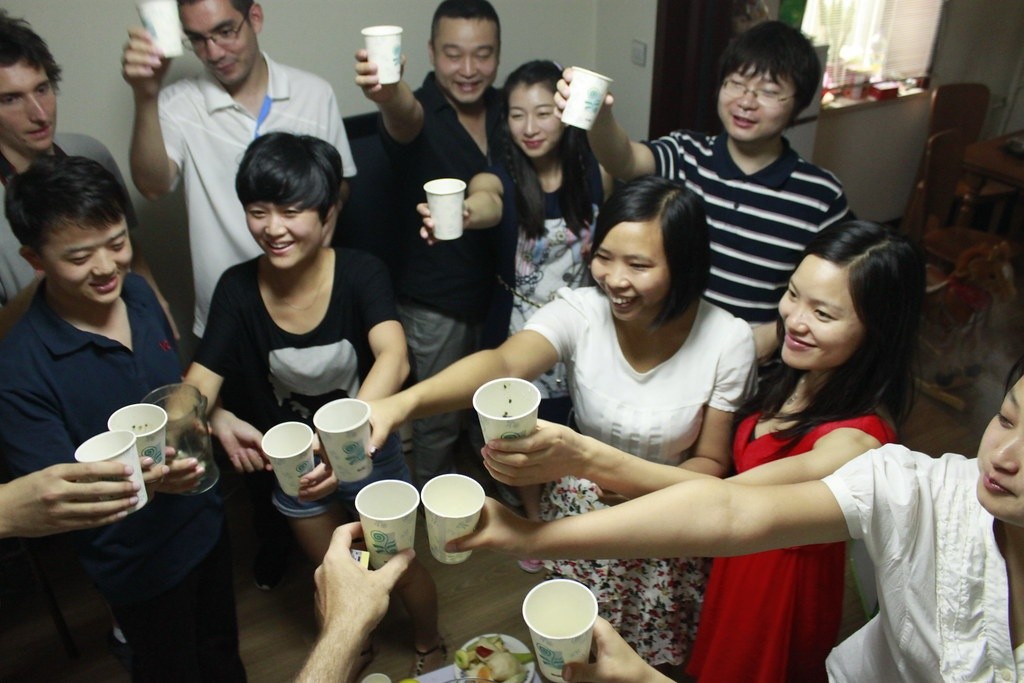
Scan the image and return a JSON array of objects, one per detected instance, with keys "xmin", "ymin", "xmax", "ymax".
[{"xmin": 871, "ymin": 81, "xmax": 898, "ymax": 101}]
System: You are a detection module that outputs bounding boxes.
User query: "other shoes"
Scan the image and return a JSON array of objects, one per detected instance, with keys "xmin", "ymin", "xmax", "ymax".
[{"xmin": 251, "ymin": 554, "xmax": 288, "ymax": 594}]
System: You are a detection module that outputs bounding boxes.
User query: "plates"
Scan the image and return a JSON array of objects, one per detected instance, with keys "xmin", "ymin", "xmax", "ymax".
[{"xmin": 454, "ymin": 635, "xmax": 537, "ymax": 683}]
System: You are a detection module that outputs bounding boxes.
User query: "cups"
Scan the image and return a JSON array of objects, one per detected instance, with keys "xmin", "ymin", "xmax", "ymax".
[
  {"xmin": 521, "ymin": 579, "xmax": 599, "ymax": 683},
  {"xmin": 260, "ymin": 421, "xmax": 317, "ymax": 498},
  {"xmin": 443, "ymin": 677, "xmax": 501, "ymax": 683},
  {"xmin": 355, "ymin": 479, "xmax": 420, "ymax": 570},
  {"xmin": 139, "ymin": 382, "xmax": 221, "ymax": 496},
  {"xmin": 107, "ymin": 403, "xmax": 168, "ymax": 486},
  {"xmin": 314, "ymin": 398, "xmax": 374, "ymax": 483},
  {"xmin": 420, "ymin": 473, "xmax": 486, "ymax": 565},
  {"xmin": 424, "ymin": 178, "xmax": 467, "ymax": 240},
  {"xmin": 560, "ymin": 66, "xmax": 614, "ymax": 132},
  {"xmin": 361, "ymin": 26, "xmax": 405, "ymax": 84},
  {"xmin": 134, "ymin": 0, "xmax": 185, "ymax": 58},
  {"xmin": 360, "ymin": 673, "xmax": 392, "ymax": 683},
  {"xmin": 74, "ymin": 430, "xmax": 148, "ymax": 517},
  {"xmin": 472, "ymin": 378, "xmax": 542, "ymax": 445}
]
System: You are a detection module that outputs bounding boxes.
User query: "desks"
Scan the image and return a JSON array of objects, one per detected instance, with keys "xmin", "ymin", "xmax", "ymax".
[{"xmin": 963, "ymin": 130, "xmax": 1024, "ymax": 189}]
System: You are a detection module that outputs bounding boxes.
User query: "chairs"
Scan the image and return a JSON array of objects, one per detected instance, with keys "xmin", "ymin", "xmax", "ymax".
[{"xmin": 903, "ymin": 82, "xmax": 1024, "ymax": 276}]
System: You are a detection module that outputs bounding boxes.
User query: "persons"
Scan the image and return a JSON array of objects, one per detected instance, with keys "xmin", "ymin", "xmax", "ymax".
[
  {"xmin": 553, "ymin": 19, "xmax": 860, "ymax": 389},
  {"xmin": 441, "ymin": 350, "xmax": 1023, "ymax": 683},
  {"xmin": 478, "ymin": 217, "xmax": 926, "ymax": 683},
  {"xmin": 292, "ymin": 521, "xmax": 680, "ymax": 683},
  {"xmin": 350, "ymin": 0, "xmax": 618, "ymax": 518},
  {"xmin": 0, "ymin": 1, "xmax": 275, "ymax": 682},
  {"xmin": 119, "ymin": 1, "xmax": 448, "ymax": 683},
  {"xmin": 365, "ymin": 173, "xmax": 756, "ymax": 671}
]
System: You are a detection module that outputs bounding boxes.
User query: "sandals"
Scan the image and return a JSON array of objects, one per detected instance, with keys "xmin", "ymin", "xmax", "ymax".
[
  {"xmin": 347, "ymin": 642, "xmax": 375, "ymax": 682},
  {"xmin": 409, "ymin": 638, "xmax": 448, "ymax": 679}
]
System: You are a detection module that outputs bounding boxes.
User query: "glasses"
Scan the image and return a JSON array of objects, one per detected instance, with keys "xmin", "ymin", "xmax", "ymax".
[
  {"xmin": 181, "ymin": 10, "xmax": 249, "ymax": 51},
  {"xmin": 721, "ymin": 78, "xmax": 799, "ymax": 106}
]
